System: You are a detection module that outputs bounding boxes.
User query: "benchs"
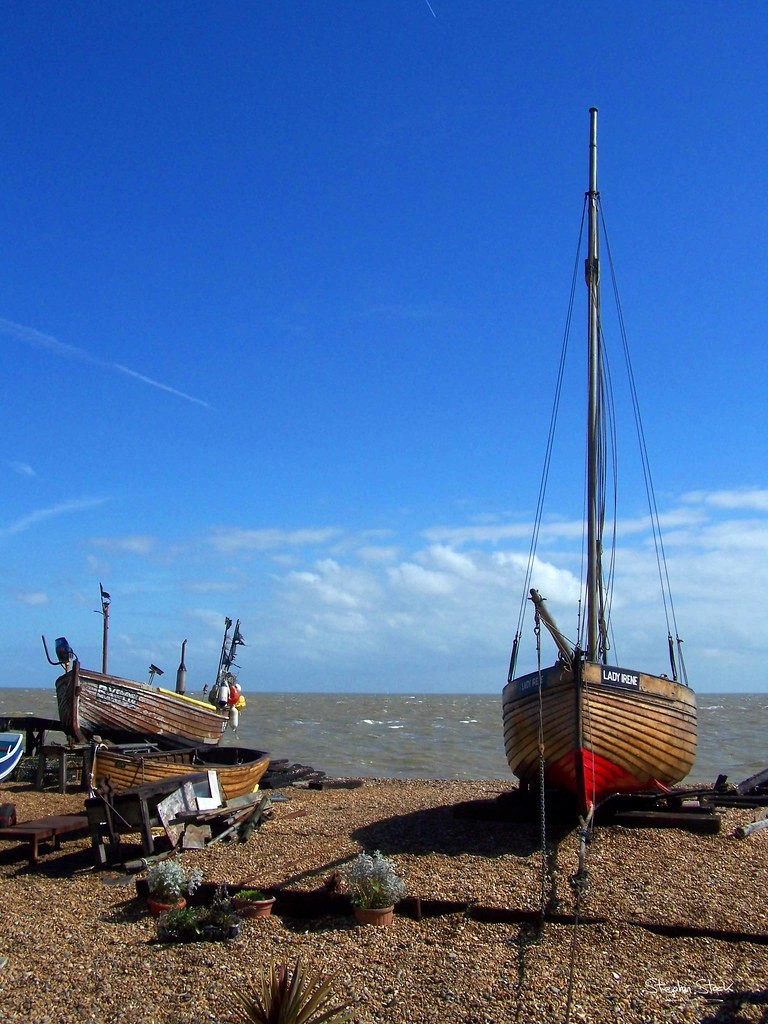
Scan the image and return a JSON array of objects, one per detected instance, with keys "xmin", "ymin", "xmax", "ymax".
[{"xmin": 0, "ymin": 815, "xmax": 90, "ymax": 866}]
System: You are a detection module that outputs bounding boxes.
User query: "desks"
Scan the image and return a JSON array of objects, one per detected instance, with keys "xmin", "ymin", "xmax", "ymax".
[
  {"xmin": 34, "ymin": 743, "xmax": 158, "ymax": 794},
  {"xmin": 84, "ymin": 770, "xmax": 224, "ymax": 862}
]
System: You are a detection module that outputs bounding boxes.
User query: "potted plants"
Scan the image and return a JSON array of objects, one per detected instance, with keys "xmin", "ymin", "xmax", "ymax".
[
  {"xmin": 144, "ymin": 858, "xmax": 204, "ymax": 919},
  {"xmin": 230, "ymin": 889, "xmax": 276, "ymax": 919},
  {"xmin": 156, "ymin": 881, "xmax": 243, "ymax": 944},
  {"xmin": 342, "ymin": 849, "xmax": 407, "ymax": 926}
]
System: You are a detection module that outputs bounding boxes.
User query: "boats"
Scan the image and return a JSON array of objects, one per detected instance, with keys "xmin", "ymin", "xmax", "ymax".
[
  {"xmin": 84, "ymin": 745, "xmax": 270, "ymax": 803},
  {"xmin": 40, "ymin": 582, "xmax": 247, "ymax": 746}
]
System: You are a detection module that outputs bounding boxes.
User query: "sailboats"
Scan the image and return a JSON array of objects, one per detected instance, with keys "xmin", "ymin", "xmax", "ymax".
[{"xmin": 503, "ymin": 106, "xmax": 699, "ymax": 814}]
